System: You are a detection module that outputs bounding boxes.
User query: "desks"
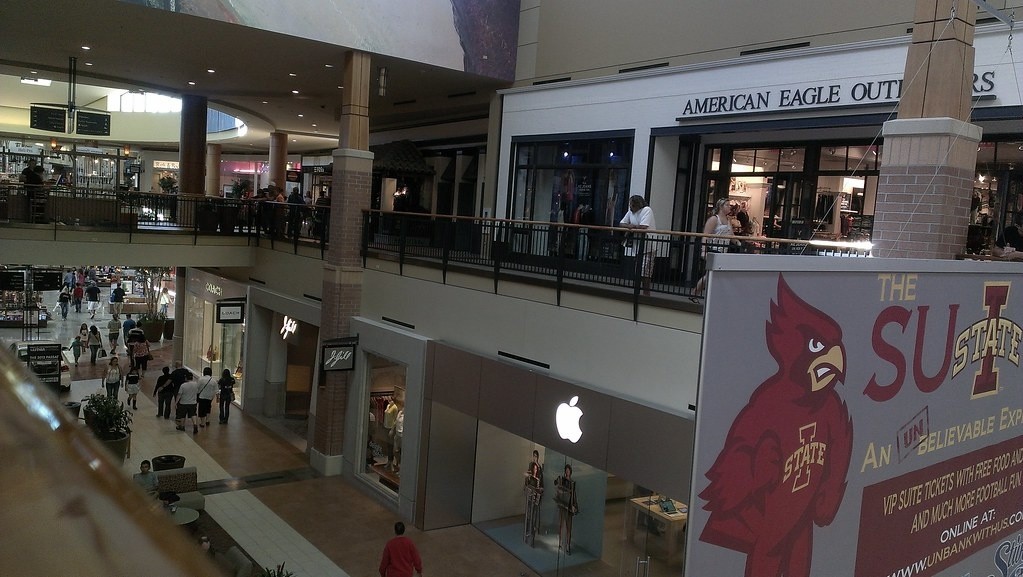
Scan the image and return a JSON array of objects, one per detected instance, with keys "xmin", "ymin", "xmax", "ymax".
[
  {"xmin": 163, "ymin": 506, "xmax": 200, "ymax": 528},
  {"xmin": 627, "ymin": 493, "xmax": 689, "ymax": 566}
]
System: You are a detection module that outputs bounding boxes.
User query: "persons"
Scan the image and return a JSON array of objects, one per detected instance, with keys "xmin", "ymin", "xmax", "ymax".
[
  {"xmin": 727, "ymin": 197, "xmax": 752, "ymax": 233},
  {"xmin": 552, "ymin": 464, "xmax": 579, "ymax": 554},
  {"xmin": 20, "ymin": 159, "xmax": 46, "ymax": 222},
  {"xmin": 559, "ymin": 170, "xmax": 577, "ymax": 224},
  {"xmin": 524, "ymin": 450, "xmax": 544, "ymax": 547},
  {"xmin": 151, "ymin": 361, "xmax": 235, "ymax": 434},
  {"xmin": 638, "ymin": 485, "xmax": 662, "ymax": 538},
  {"xmin": 991, "ymin": 210, "xmax": 1022, "ymax": 260},
  {"xmin": 605, "ymin": 169, "xmax": 620, "ymax": 236},
  {"xmin": 239, "ymin": 180, "xmax": 330, "ymax": 243},
  {"xmin": 69, "ymin": 313, "xmax": 152, "ymax": 378},
  {"xmin": 156, "ymin": 287, "xmax": 173, "ymax": 315},
  {"xmin": 617, "ymin": 195, "xmax": 657, "ymax": 296},
  {"xmin": 379, "ymin": 521, "xmax": 424, "ymax": 577},
  {"xmin": 689, "ymin": 198, "xmax": 742, "ymax": 305},
  {"xmin": 383, "ymin": 401, "xmax": 405, "ymax": 477},
  {"xmin": 970, "ymin": 191, "xmax": 980, "ymax": 224},
  {"xmin": 197, "ymin": 536, "xmax": 216, "ymax": 560},
  {"xmin": 99, "ymin": 357, "xmax": 144, "ymax": 410},
  {"xmin": 58, "ymin": 265, "xmax": 127, "ymax": 318},
  {"xmin": 133, "ymin": 459, "xmax": 158, "ymax": 502}
]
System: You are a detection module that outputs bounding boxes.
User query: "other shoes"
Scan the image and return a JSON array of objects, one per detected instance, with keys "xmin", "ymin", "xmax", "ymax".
[
  {"xmin": 156, "ymin": 414, "xmax": 170, "ymax": 420},
  {"xmin": 219, "ymin": 421, "xmax": 229, "ymax": 424},
  {"xmin": 200, "ymin": 423, "xmax": 204, "ymax": 427},
  {"xmin": 206, "ymin": 421, "xmax": 210, "ymax": 425}
]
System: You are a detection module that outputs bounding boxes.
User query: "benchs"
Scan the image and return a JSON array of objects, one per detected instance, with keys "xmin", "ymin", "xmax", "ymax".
[
  {"xmin": 133, "ymin": 465, "xmax": 206, "ymax": 511},
  {"xmin": 213, "ymin": 545, "xmax": 253, "ymax": 577}
]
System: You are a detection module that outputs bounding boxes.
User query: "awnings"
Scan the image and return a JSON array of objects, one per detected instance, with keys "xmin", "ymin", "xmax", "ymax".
[{"xmin": 328, "ymin": 140, "xmax": 437, "ymax": 172}]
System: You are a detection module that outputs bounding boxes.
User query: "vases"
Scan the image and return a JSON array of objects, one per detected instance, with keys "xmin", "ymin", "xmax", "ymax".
[
  {"xmin": 162, "ymin": 317, "xmax": 175, "ymax": 340},
  {"xmin": 196, "ymin": 203, "xmax": 241, "ymax": 234}
]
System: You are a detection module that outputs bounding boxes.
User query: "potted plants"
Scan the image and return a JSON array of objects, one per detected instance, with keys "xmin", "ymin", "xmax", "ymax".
[
  {"xmin": 62, "ymin": 392, "xmax": 134, "ymax": 466},
  {"xmin": 130, "ymin": 265, "xmax": 171, "ymax": 343}
]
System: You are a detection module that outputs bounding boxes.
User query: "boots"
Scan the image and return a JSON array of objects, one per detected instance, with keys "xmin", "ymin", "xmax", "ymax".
[
  {"xmin": 133, "ymin": 400, "xmax": 137, "ymax": 410},
  {"xmin": 127, "ymin": 398, "xmax": 131, "ymax": 407}
]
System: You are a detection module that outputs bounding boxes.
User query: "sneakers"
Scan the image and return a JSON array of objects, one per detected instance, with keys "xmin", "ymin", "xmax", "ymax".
[
  {"xmin": 176, "ymin": 425, "xmax": 185, "ymax": 431},
  {"xmin": 688, "ymin": 294, "xmax": 700, "ymax": 305},
  {"xmin": 194, "ymin": 428, "xmax": 198, "ymax": 433}
]
